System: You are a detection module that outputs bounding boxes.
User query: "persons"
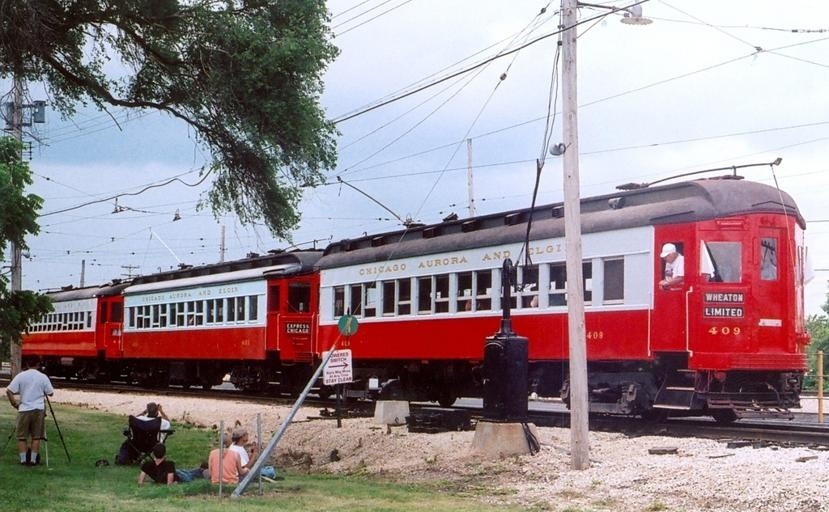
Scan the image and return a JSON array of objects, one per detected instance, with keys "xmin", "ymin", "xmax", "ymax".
[
  {"xmin": 227, "ymin": 425, "xmax": 276, "ymax": 482},
  {"xmin": 203, "ymin": 431, "xmax": 250, "ymax": 485},
  {"xmin": 657, "ymin": 244, "xmax": 684, "ymax": 291},
  {"xmin": 138, "ymin": 444, "xmax": 207, "ymax": 489},
  {"xmin": 465, "ymin": 291, "xmax": 481, "ymax": 311},
  {"xmin": 529, "ymin": 295, "xmax": 537, "ymax": 307},
  {"xmin": 6, "ymin": 353, "xmax": 54, "ymax": 466},
  {"xmin": 115, "ymin": 403, "xmax": 172, "ymax": 462}
]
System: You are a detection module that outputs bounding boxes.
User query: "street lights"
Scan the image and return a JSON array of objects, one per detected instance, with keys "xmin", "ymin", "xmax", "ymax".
[{"xmin": 556, "ymin": 0, "xmax": 655, "ymax": 465}]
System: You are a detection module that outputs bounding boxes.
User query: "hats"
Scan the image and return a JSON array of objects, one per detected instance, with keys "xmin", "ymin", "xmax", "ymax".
[{"xmin": 232, "ymin": 428, "xmax": 247, "ymax": 439}]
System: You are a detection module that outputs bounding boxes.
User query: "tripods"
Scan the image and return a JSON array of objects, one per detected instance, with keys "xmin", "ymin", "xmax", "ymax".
[{"xmin": 0, "ymin": 391, "xmax": 73, "ymax": 465}]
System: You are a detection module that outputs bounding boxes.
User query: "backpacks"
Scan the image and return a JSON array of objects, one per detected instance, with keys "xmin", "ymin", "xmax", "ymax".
[{"xmin": 116, "ymin": 438, "xmax": 141, "ymax": 465}]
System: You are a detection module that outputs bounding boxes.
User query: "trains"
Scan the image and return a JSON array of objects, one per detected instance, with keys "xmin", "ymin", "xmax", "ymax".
[{"xmin": 21, "ymin": 157, "xmax": 814, "ymax": 423}]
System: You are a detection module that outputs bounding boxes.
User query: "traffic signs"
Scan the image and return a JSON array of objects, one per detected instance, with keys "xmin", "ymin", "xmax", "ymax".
[{"xmin": 321, "ymin": 349, "xmax": 353, "ymax": 385}]
